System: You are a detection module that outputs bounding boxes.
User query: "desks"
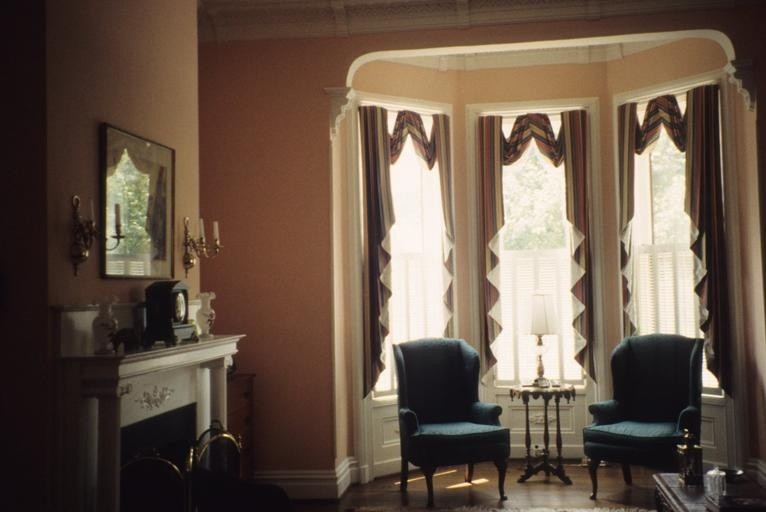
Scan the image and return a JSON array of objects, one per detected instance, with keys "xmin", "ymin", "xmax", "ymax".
[
  {"xmin": 649, "ymin": 469, "xmax": 765, "ymax": 512},
  {"xmin": 509, "ymin": 382, "xmax": 577, "ymax": 487}
]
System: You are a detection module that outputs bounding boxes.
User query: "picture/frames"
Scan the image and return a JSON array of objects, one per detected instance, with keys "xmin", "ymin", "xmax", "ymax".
[{"xmin": 97, "ymin": 118, "xmax": 178, "ymax": 285}]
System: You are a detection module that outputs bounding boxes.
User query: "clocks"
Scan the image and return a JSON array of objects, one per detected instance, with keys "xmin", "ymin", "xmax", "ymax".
[{"xmin": 135, "ymin": 278, "xmax": 199, "ymax": 350}]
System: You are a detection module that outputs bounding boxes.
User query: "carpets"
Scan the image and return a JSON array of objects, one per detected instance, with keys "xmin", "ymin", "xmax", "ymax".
[{"xmin": 344, "ymin": 503, "xmax": 656, "ymax": 511}]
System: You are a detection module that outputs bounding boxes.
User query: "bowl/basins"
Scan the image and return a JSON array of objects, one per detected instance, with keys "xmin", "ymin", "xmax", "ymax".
[{"xmin": 718, "ymin": 466, "xmax": 746, "ymax": 480}]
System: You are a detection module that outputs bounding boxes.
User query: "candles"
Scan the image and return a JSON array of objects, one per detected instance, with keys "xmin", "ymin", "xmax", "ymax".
[
  {"xmin": 196, "ymin": 217, "xmax": 205, "ymax": 240},
  {"xmin": 87, "ymin": 196, "xmax": 95, "ymax": 222},
  {"xmin": 113, "ymin": 200, "xmax": 122, "ymax": 229},
  {"xmin": 210, "ymin": 218, "xmax": 219, "ymax": 242}
]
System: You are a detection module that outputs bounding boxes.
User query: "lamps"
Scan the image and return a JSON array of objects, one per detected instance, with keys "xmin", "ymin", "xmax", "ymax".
[{"xmin": 522, "ymin": 294, "xmax": 563, "ymax": 390}]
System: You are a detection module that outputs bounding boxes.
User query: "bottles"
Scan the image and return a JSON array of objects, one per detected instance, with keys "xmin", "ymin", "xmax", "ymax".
[{"xmin": 132, "ymin": 301, "xmax": 147, "ymax": 345}]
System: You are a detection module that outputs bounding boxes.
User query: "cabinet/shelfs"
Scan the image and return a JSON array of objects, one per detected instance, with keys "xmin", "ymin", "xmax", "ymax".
[{"xmin": 219, "ymin": 374, "xmax": 257, "ymax": 477}]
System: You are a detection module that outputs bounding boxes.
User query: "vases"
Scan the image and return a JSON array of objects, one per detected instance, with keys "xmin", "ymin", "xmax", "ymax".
[
  {"xmin": 192, "ymin": 290, "xmax": 218, "ymax": 340},
  {"xmin": 87, "ymin": 293, "xmax": 121, "ymax": 359}
]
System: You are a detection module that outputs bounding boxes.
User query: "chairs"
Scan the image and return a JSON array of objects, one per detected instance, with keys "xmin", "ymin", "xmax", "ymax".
[
  {"xmin": 391, "ymin": 334, "xmax": 513, "ymax": 510},
  {"xmin": 581, "ymin": 333, "xmax": 705, "ymax": 506}
]
